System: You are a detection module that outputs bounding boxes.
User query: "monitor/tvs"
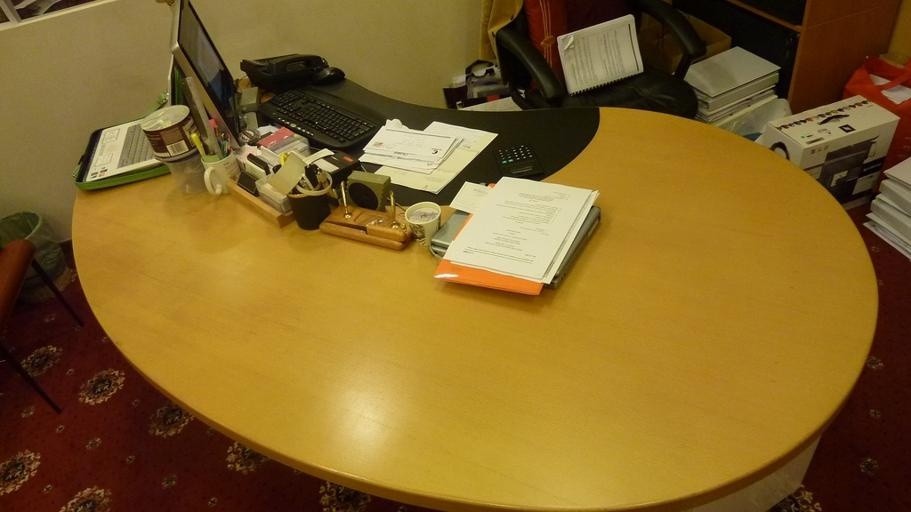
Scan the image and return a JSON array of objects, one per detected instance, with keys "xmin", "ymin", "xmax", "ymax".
[{"xmin": 169, "ymin": 0, "xmax": 241, "ymax": 151}]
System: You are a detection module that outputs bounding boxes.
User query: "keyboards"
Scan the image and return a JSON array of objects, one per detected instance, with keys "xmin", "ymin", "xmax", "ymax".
[{"xmin": 260, "ymin": 87, "xmax": 384, "ymax": 149}]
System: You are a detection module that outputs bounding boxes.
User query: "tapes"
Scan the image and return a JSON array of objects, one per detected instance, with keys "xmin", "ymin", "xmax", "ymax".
[{"xmin": 293, "ymin": 166, "xmax": 335, "ymax": 197}]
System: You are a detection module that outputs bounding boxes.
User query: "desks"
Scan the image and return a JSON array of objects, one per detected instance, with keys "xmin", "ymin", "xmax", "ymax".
[{"xmin": 68, "ymin": 54, "xmax": 882, "ymax": 512}]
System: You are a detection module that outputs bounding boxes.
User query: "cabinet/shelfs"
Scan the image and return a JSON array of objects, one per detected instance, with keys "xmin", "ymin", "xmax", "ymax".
[{"xmin": 562, "ymin": 0, "xmax": 902, "ymax": 114}]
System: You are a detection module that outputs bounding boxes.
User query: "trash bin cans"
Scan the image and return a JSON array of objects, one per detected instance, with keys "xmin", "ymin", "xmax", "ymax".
[{"xmin": 1, "ymin": 210, "xmax": 66, "ymax": 276}]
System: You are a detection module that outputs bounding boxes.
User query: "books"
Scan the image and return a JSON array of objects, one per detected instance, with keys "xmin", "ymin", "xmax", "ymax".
[
  {"xmin": 557, "ymin": 14, "xmax": 644, "ymax": 95},
  {"xmin": 863, "ymin": 156, "xmax": 911, "ymax": 262},
  {"xmin": 684, "ymin": 46, "xmax": 781, "ymax": 126}
]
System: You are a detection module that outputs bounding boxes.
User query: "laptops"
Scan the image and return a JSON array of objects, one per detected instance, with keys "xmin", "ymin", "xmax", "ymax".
[{"xmin": 76, "ymin": 50, "xmax": 174, "ymax": 182}]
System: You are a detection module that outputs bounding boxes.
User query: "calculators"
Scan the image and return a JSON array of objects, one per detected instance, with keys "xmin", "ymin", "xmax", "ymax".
[{"xmin": 494, "ymin": 143, "xmax": 544, "ymax": 177}]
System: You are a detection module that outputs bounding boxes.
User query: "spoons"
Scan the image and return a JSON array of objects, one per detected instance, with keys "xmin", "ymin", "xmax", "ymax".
[
  {"xmin": 339, "ymin": 182, "xmax": 351, "ymax": 217},
  {"xmin": 388, "ymin": 192, "xmax": 401, "ymax": 229}
]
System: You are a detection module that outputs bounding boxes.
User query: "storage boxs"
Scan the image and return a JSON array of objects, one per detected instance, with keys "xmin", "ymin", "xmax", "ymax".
[{"xmin": 761, "ymin": 96, "xmax": 901, "ymax": 212}]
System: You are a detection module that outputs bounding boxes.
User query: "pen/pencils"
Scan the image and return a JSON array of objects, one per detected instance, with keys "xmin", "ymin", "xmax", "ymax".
[{"xmin": 191, "ymin": 120, "xmax": 231, "ymax": 161}]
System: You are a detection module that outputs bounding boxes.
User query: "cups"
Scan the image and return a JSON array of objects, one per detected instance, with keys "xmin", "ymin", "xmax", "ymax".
[
  {"xmin": 404, "ymin": 202, "xmax": 442, "ymax": 246},
  {"xmin": 292, "ymin": 171, "xmax": 330, "ymax": 230},
  {"xmin": 201, "ymin": 149, "xmax": 241, "ymax": 194}
]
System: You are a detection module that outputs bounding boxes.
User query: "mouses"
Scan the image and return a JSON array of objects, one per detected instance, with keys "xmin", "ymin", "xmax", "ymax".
[{"xmin": 316, "ymin": 67, "xmax": 345, "ymax": 84}]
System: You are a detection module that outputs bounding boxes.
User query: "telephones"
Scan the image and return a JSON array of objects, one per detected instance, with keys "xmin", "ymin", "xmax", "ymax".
[{"xmin": 240, "ymin": 53, "xmax": 330, "ymax": 88}]
